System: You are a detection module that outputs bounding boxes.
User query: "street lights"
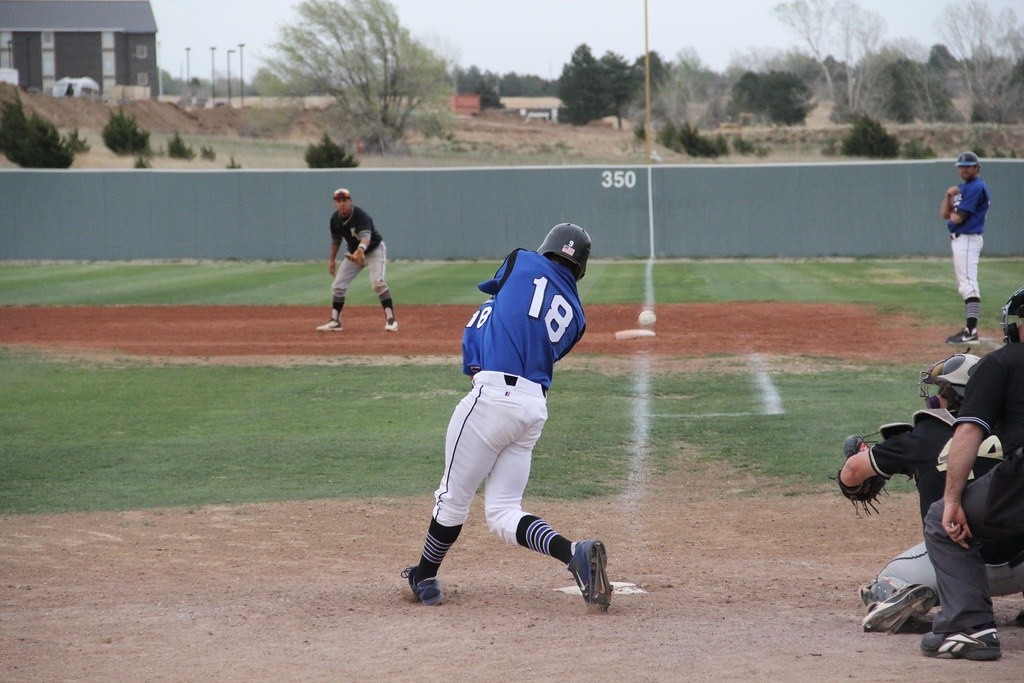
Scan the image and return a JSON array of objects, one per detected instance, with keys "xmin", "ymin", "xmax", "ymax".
[
  {"xmin": 185, "ymin": 47, "xmax": 190, "ymax": 83},
  {"xmin": 237, "ymin": 43, "xmax": 246, "ymax": 108},
  {"xmin": 226, "ymin": 49, "xmax": 236, "ymax": 108},
  {"xmin": 209, "ymin": 47, "xmax": 216, "ymax": 107}
]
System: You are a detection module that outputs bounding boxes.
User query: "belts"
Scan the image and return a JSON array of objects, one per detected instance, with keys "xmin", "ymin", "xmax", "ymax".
[
  {"xmin": 950, "ymin": 232, "xmax": 960, "ymax": 239},
  {"xmin": 503, "ymin": 375, "xmax": 546, "ymax": 398}
]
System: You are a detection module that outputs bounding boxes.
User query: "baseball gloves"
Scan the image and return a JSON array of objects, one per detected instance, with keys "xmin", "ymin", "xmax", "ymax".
[
  {"xmin": 344, "ymin": 248, "xmax": 365, "ymax": 266},
  {"xmin": 837, "ymin": 434, "xmax": 887, "ymax": 502}
]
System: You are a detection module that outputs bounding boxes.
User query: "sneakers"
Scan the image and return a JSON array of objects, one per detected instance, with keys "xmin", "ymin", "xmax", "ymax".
[
  {"xmin": 401, "ymin": 566, "xmax": 443, "ymax": 605},
  {"xmin": 384, "ymin": 320, "xmax": 397, "ymax": 332},
  {"xmin": 863, "ymin": 584, "xmax": 937, "ymax": 634},
  {"xmin": 945, "ymin": 327, "xmax": 979, "ymax": 344},
  {"xmin": 922, "ymin": 623, "xmax": 1002, "ymax": 661},
  {"xmin": 567, "ymin": 538, "xmax": 614, "ymax": 611},
  {"xmin": 316, "ymin": 320, "xmax": 343, "ymax": 331}
]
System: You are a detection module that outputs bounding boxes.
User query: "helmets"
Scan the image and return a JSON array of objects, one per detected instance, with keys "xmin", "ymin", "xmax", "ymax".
[
  {"xmin": 954, "ymin": 151, "xmax": 979, "ymax": 166},
  {"xmin": 940, "ymin": 353, "xmax": 982, "ymax": 402},
  {"xmin": 536, "ymin": 222, "xmax": 591, "ymax": 282}
]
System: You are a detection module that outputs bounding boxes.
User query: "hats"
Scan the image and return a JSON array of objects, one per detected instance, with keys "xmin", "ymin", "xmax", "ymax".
[{"xmin": 333, "ymin": 188, "xmax": 350, "ymax": 200}]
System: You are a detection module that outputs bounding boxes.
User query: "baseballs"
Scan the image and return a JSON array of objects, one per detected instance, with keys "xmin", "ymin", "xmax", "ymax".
[{"xmin": 639, "ymin": 310, "xmax": 657, "ymax": 327}]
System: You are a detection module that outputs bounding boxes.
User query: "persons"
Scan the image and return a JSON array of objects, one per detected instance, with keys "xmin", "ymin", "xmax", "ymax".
[
  {"xmin": 919, "ymin": 284, "xmax": 1024, "ymax": 659},
  {"xmin": 828, "ymin": 352, "xmax": 1024, "ymax": 635},
  {"xmin": 315, "ymin": 188, "xmax": 399, "ymax": 332},
  {"xmin": 398, "ymin": 222, "xmax": 613, "ymax": 610},
  {"xmin": 942, "ymin": 151, "xmax": 990, "ymax": 347}
]
